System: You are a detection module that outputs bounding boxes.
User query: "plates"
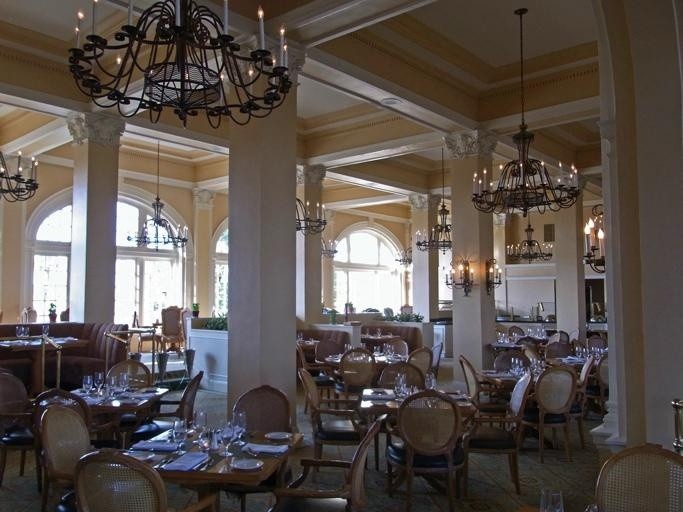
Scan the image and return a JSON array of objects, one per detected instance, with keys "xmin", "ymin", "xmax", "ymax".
[
  {"xmin": 264, "ymin": 431, "xmax": 291, "ymax": 439},
  {"xmin": 324, "ymin": 353, "xmax": 408, "ymax": 363},
  {"xmin": 370, "ymin": 383, "xmax": 388, "ymax": 407},
  {"xmin": 229, "ymin": 458, "xmax": 264, "ymax": 469},
  {"xmin": 481, "ymin": 370, "xmax": 511, "ymax": 378},
  {"xmin": 121, "ymin": 450, "xmax": 155, "ymax": 461},
  {"xmin": 1, "ymin": 337, "xmax": 77, "ymax": 345}
]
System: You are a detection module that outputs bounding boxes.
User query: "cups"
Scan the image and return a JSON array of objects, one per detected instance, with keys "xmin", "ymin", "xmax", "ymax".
[{"xmin": 539, "ymin": 488, "xmax": 564, "ymax": 512}]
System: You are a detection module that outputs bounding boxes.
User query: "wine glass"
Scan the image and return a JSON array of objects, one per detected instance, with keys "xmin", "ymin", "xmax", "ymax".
[
  {"xmin": 394, "ymin": 372, "xmax": 435, "ymax": 402},
  {"xmin": 163, "ymin": 406, "xmax": 248, "ymax": 460},
  {"xmin": 75, "ymin": 369, "xmax": 158, "ymax": 409},
  {"xmin": 364, "ymin": 326, "xmax": 392, "ymax": 339},
  {"xmin": 15, "ymin": 325, "xmax": 49, "ymax": 340},
  {"xmin": 575, "ymin": 347, "xmax": 604, "ymax": 361},
  {"xmin": 510, "ymin": 358, "xmax": 545, "ymax": 376},
  {"xmin": 496, "ymin": 326, "xmax": 547, "ymax": 344},
  {"xmin": 344, "ymin": 342, "xmax": 394, "ymax": 363}
]
